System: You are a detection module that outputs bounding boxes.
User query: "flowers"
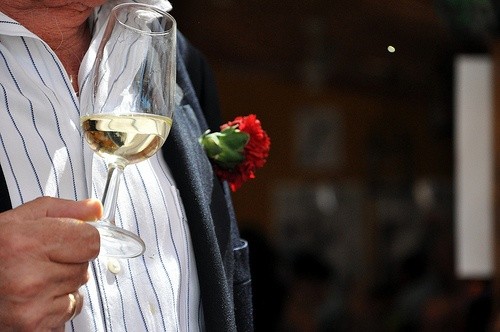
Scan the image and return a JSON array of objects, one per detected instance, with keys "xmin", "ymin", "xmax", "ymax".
[{"xmin": 197, "ymin": 113, "xmax": 271, "ymax": 195}]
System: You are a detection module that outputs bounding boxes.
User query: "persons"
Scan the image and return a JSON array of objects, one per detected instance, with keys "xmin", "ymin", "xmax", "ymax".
[{"xmin": 1, "ymin": 1, "xmax": 255, "ymax": 332}]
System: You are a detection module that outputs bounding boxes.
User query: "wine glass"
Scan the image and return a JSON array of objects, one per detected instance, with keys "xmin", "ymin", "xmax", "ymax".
[{"xmin": 78, "ymin": 3, "xmax": 178, "ymax": 259}]
